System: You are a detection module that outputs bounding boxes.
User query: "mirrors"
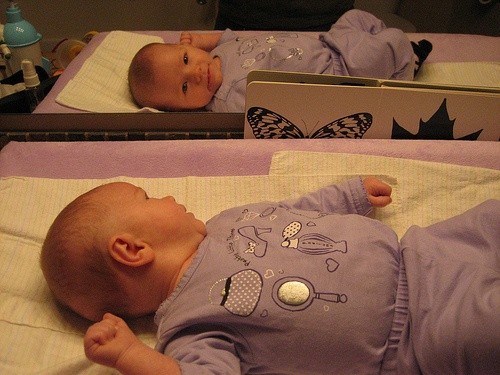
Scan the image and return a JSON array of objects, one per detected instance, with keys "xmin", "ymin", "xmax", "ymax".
[{"xmin": 0, "ymin": 0, "xmax": 500, "ymax": 142}]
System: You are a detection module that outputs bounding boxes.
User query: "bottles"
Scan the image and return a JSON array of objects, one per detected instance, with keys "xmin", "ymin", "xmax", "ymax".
[
  {"xmin": 2, "ymin": 6, "xmax": 44, "ymax": 74},
  {"xmin": 53, "ymin": 38, "xmax": 86, "ymax": 69}
]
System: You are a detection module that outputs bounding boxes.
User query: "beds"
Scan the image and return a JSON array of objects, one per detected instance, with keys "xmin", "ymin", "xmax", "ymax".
[
  {"xmin": 31, "ymin": 31, "xmax": 500, "ymax": 113},
  {"xmin": 0, "ymin": 132, "xmax": 500, "ymax": 375}
]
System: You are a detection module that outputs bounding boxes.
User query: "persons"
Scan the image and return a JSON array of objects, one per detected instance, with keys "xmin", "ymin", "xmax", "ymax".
[
  {"xmin": 128, "ymin": 10, "xmax": 433, "ymax": 112},
  {"xmin": 39, "ymin": 176, "xmax": 500, "ymax": 375}
]
